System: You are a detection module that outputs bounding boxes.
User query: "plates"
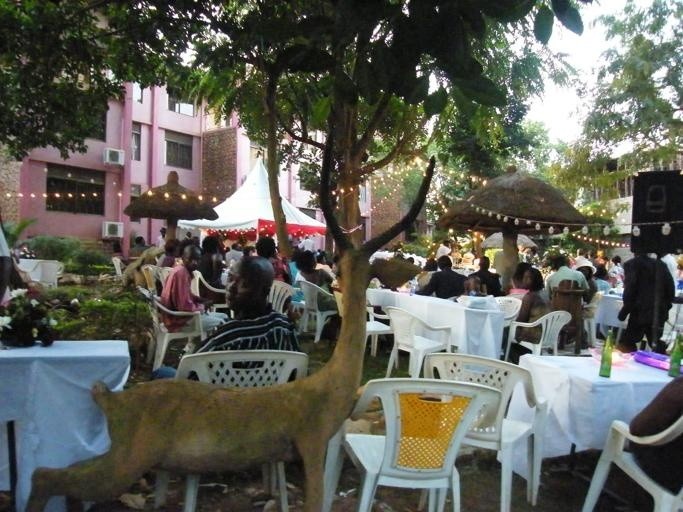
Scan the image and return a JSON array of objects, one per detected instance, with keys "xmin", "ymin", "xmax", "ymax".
[{"xmin": 588, "ymin": 347, "xmax": 631, "ymax": 366}]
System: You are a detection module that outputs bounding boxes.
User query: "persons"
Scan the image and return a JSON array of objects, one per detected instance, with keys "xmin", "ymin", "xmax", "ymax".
[
  {"xmin": 554, "ymin": 371, "xmax": 683, "ymax": 512},
  {"xmin": 197, "ymin": 236, "xmax": 229, "ymax": 303},
  {"xmin": 617, "ymin": 245, "xmax": 674, "ymax": 355},
  {"xmin": 0, "ymin": 226, "xmax": 14, "ymax": 310},
  {"xmin": 504, "ymin": 268, "xmax": 550, "ymax": 364},
  {"xmin": 225, "ymin": 242, "xmax": 244, "ymax": 263},
  {"xmin": 153, "ymin": 239, "xmax": 180, "ymax": 297},
  {"xmin": 128, "ymin": 237, "xmax": 151, "ymax": 264},
  {"xmin": 296, "ymin": 249, "xmax": 338, "ymax": 338},
  {"xmin": 256, "ymin": 237, "xmax": 293, "ymax": 313},
  {"xmin": 435, "ymin": 240, "xmax": 453, "ymax": 262},
  {"xmin": 418, "ymin": 258, "xmax": 439, "ymax": 289},
  {"xmin": 571, "ymin": 256, "xmax": 597, "ymax": 302},
  {"xmin": 314, "ymin": 256, "xmax": 335, "ymax": 280},
  {"xmin": 241, "ymin": 246, "xmax": 256, "ymax": 256},
  {"xmin": 159, "ymin": 245, "xmax": 230, "ymax": 359},
  {"xmin": 154, "ymin": 227, "xmax": 169, "ymax": 261},
  {"xmin": 505, "ymin": 262, "xmax": 532, "ymax": 294},
  {"xmin": 468, "ymin": 256, "xmax": 500, "ymax": 296},
  {"xmin": 415, "ymin": 255, "xmax": 467, "ymax": 298},
  {"xmin": 464, "ymin": 276, "xmax": 482, "ymax": 296},
  {"xmin": 151, "ymin": 255, "xmax": 305, "ymax": 384},
  {"xmin": 594, "ymin": 255, "xmax": 624, "ymax": 286},
  {"xmin": 180, "ymin": 232, "xmax": 198, "ymax": 249},
  {"xmin": 546, "ymin": 256, "xmax": 591, "ymax": 349}
]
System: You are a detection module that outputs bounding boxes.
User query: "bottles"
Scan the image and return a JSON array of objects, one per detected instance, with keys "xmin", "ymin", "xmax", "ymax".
[
  {"xmin": 617, "ymin": 279, "xmax": 621, "ymax": 289},
  {"xmin": 676, "ymin": 334, "xmax": 683, "ymax": 359},
  {"xmin": 410, "ymin": 277, "xmax": 416, "ymax": 296},
  {"xmin": 668, "ymin": 339, "xmax": 682, "ymax": 378},
  {"xmin": 599, "ymin": 330, "xmax": 613, "ymax": 377}
]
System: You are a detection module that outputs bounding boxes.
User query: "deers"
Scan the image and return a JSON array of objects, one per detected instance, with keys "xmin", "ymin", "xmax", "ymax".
[{"xmin": 26, "ymin": 133, "xmax": 434, "ymax": 507}]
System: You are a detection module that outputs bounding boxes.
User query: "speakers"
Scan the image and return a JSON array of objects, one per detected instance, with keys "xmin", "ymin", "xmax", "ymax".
[{"xmin": 632, "ymin": 168, "xmax": 683, "ymax": 259}]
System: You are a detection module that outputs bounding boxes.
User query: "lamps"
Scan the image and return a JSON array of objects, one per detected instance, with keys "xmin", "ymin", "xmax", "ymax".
[
  {"xmin": 497, "ymin": 214, "xmax": 501, "ymax": 220},
  {"xmin": 488, "ymin": 211, "xmax": 492, "ymax": 217},
  {"xmin": 604, "ymin": 225, "xmax": 611, "ymax": 236},
  {"xmin": 514, "ymin": 218, "xmax": 519, "ymax": 225},
  {"xmin": 581, "ymin": 225, "xmax": 589, "ymax": 234},
  {"xmin": 548, "ymin": 226, "xmax": 554, "ymax": 234},
  {"xmin": 504, "ymin": 216, "xmax": 508, "ymax": 222},
  {"xmin": 633, "ymin": 226, "xmax": 640, "ymax": 236},
  {"xmin": 476, "ymin": 207, "xmax": 479, "ymax": 212},
  {"xmin": 536, "ymin": 223, "xmax": 540, "ymax": 230},
  {"xmin": 563, "ymin": 226, "xmax": 570, "ymax": 235},
  {"xmin": 482, "ymin": 209, "xmax": 485, "ymax": 214},
  {"xmin": 661, "ymin": 223, "xmax": 670, "ymax": 235}
]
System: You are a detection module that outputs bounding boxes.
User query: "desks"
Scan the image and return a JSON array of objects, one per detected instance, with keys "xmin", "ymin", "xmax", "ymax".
[{"xmin": 0, "ymin": 341, "xmax": 131, "ymax": 512}]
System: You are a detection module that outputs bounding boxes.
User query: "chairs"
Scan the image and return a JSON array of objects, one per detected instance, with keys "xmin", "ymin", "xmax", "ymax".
[
  {"xmin": 423, "ymin": 352, "xmax": 549, "ymax": 512},
  {"xmin": 504, "ymin": 311, "xmax": 572, "ymax": 363},
  {"xmin": 295, "ymin": 280, "xmax": 340, "ymax": 344},
  {"xmin": 137, "ymin": 285, "xmax": 207, "ymax": 372},
  {"xmin": 385, "ymin": 305, "xmax": 453, "ymax": 380},
  {"xmin": 549, "ymin": 286, "xmax": 588, "ymax": 355},
  {"xmin": 156, "ymin": 267, "xmax": 171, "ymax": 287},
  {"xmin": 333, "ymin": 291, "xmax": 392, "ymax": 357},
  {"xmin": 580, "ymin": 414, "xmax": 683, "ymax": 512},
  {"xmin": 155, "ymin": 348, "xmax": 308, "ymax": 512},
  {"xmin": 140, "ymin": 264, "xmax": 158, "ymax": 296},
  {"xmin": 112, "ymin": 258, "xmax": 127, "ymax": 277},
  {"xmin": 192, "ymin": 271, "xmax": 235, "ymax": 319},
  {"xmin": 267, "ymin": 280, "xmax": 305, "ymax": 318},
  {"xmin": 321, "ymin": 378, "xmax": 502, "ymax": 512},
  {"xmin": 485, "ymin": 295, "xmax": 523, "ymax": 336}
]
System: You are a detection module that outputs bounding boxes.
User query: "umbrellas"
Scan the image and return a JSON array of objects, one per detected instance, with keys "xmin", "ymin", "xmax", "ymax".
[
  {"xmin": 124, "ymin": 171, "xmax": 219, "ymax": 243},
  {"xmin": 432, "ymin": 167, "xmax": 589, "ymax": 263},
  {"xmin": 177, "ymin": 156, "xmax": 328, "ymax": 240},
  {"xmin": 600, "ymin": 234, "xmax": 633, "ymax": 248}
]
